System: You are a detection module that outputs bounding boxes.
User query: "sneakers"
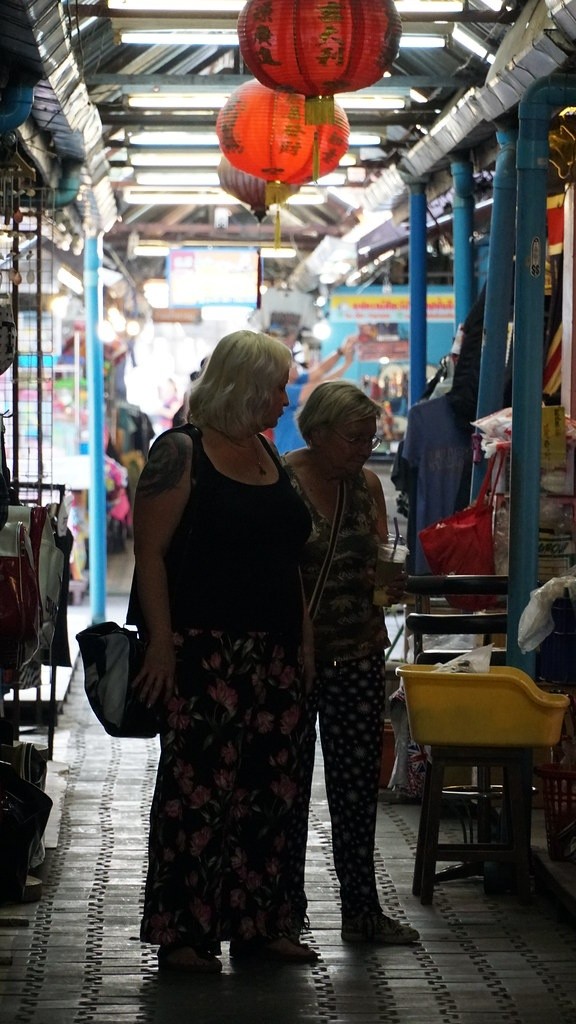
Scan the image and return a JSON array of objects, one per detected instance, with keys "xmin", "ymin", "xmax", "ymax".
[{"xmin": 342, "ymin": 913, "xmax": 420, "ymax": 942}]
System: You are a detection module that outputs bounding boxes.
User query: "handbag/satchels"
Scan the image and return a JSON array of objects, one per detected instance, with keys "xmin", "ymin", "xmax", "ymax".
[
  {"xmin": 75, "ymin": 622, "xmax": 167, "ymax": 738},
  {"xmin": 417, "ymin": 442, "xmax": 508, "ymax": 610},
  {"xmin": 0, "ymin": 504, "xmax": 72, "ymax": 667},
  {"xmin": 0, "ymin": 744, "xmax": 53, "ymax": 900}
]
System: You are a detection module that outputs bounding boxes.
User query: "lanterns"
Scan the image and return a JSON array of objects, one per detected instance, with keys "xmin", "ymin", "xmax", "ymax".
[
  {"xmin": 216, "ymin": 79, "xmax": 351, "ymax": 208},
  {"xmin": 218, "ymin": 157, "xmax": 300, "ymax": 222},
  {"xmin": 237, "ymin": 0, "xmax": 402, "ymax": 126}
]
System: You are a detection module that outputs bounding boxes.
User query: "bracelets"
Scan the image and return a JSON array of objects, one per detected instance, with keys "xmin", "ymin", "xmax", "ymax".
[{"xmin": 337, "ymin": 349, "xmax": 343, "ymax": 355}]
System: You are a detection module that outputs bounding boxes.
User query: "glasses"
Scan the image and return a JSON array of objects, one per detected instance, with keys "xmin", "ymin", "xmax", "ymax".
[{"xmin": 326, "ymin": 424, "xmax": 383, "ymax": 451}]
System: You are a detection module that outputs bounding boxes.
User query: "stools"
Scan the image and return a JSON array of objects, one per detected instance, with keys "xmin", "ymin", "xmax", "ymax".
[{"xmin": 413, "ymin": 743, "xmax": 538, "ymax": 905}]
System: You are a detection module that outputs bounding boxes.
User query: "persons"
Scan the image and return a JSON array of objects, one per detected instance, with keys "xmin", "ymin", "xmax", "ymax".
[
  {"xmin": 157, "ymin": 378, "xmax": 182, "ymax": 431},
  {"xmin": 127, "ymin": 330, "xmax": 317, "ymax": 972},
  {"xmin": 362, "ymin": 363, "xmax": 446, "ymax": 454},
  {"xmin": 273, "ymin": 334, "xmax": 359, "ymax": 457},
  {"xmin": 281, "ymin": 383, "xmax": 419, "ymax": 949}
]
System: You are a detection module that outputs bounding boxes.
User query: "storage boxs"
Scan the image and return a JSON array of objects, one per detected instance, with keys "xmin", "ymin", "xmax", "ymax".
[{"xmin": 396, "ymin": 663, "xmax": 566, "ymax": 745}]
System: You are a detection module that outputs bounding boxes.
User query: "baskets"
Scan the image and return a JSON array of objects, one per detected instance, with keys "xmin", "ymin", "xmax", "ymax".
[{"xmin": 533, "ymin": 763, "xmax": 576, "ymax": 861}]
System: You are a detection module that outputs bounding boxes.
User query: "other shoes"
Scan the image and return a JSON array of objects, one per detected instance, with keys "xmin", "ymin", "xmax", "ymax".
[
  {"xmin": 229, "ymin": 937, "xmax": 317, "ymax": 962},
  {"xmin": 158, "ymin": 943, "xmax": 221, "ymax": 972}
]
